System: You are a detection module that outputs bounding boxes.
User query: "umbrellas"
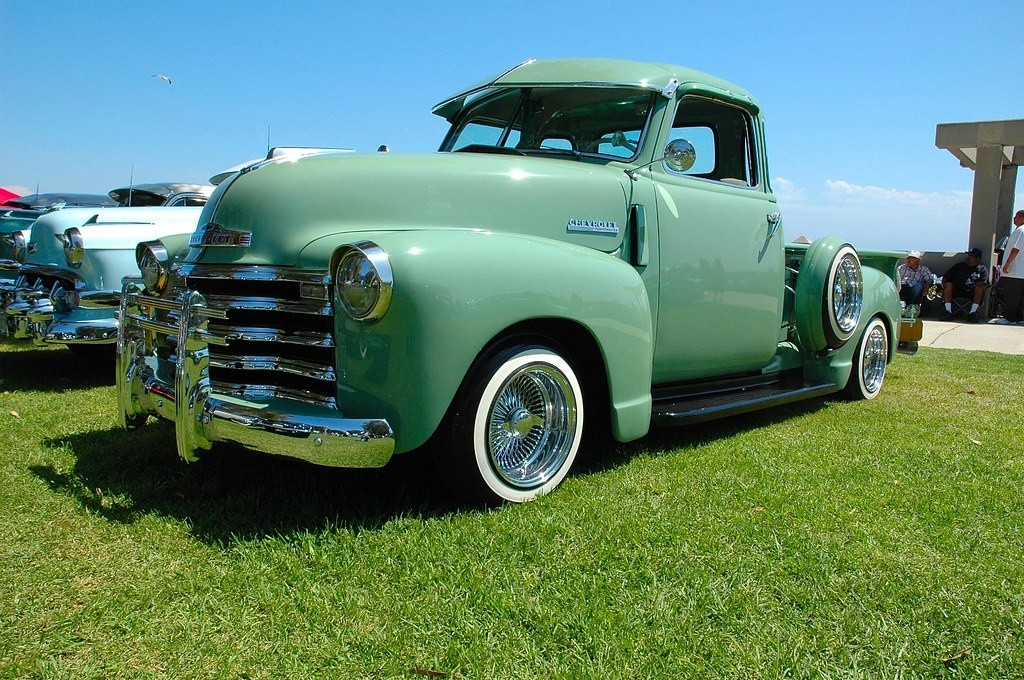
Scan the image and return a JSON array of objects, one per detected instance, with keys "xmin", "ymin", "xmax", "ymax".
[{"xmin": 0, "ymin": 188, "xmax": 23, "ymax": 210}]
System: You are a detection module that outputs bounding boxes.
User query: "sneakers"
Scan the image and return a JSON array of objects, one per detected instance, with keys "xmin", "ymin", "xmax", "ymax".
[
  {"xmin": 995, "ymin": 319, "xmax": 1023, "ymax": 324},
  {"xmin": 939, "ymin": 310, "xmax": 955, "ymax": 321},
  {"xmin": 967, "ymin": 312, "xmax": 979, "ymax": 323}
]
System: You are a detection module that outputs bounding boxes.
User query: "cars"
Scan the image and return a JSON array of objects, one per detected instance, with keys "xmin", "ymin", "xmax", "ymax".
[
  {"xmin": 112, "ymin": 55, "xmax": 925, "ymax": 504},
  {"xmin": 0, "ymin": 157, "xmax": 266, "ymax": 364}
]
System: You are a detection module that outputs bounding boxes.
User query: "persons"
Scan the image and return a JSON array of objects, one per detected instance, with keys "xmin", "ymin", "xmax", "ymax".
[
  {"xmin": 995, "ymin": 210, "xmax": 1024, "ymax": 325},
  {"xmin": 899, "ymin": 250, "xmax": 933, "ymax": 306},
  {"xmin": 942, "ymin": 248, "xmax": 989, "ymax": 323}
]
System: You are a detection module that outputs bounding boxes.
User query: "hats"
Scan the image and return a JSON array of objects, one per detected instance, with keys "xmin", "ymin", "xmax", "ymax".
[
  {"xmin": 965, "ymin": 248, "xmax": 982, "ymax": 257},
  {"xmin": 907, "ymin": 250, "xmax": 921, "ymax": 260}
]
